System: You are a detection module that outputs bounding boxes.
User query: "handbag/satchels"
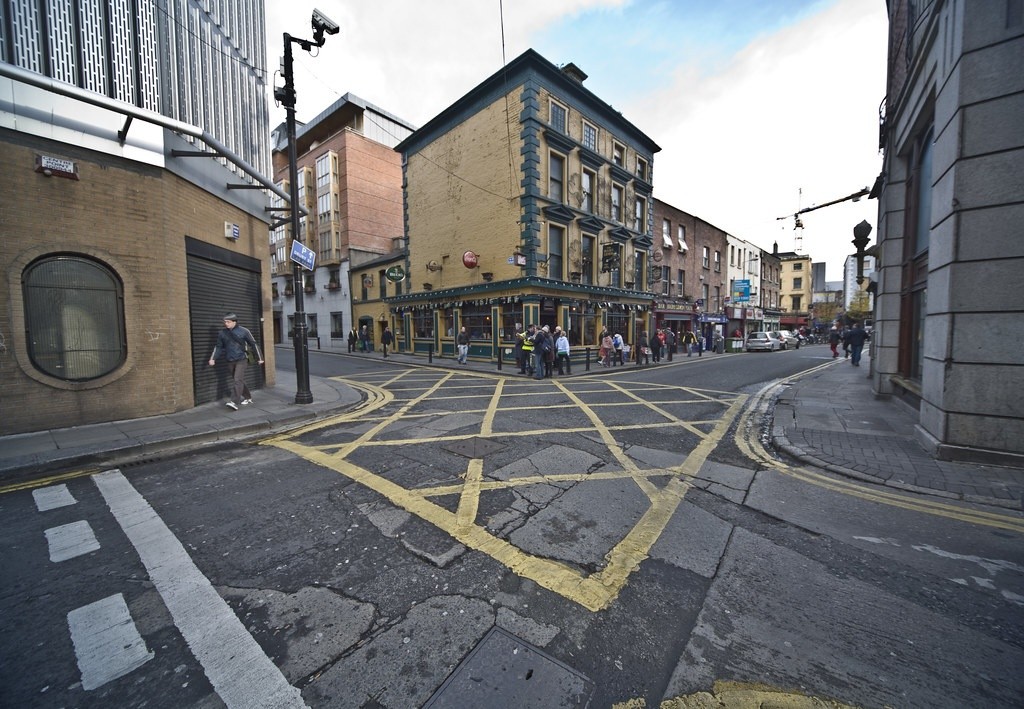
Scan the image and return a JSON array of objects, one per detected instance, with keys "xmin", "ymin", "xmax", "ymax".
[{"xmin": 245, "ymin": 349, "xmax": 257, "ymax": 364}]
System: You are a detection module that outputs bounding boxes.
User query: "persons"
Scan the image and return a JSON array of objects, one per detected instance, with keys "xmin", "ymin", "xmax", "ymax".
[
  {"xmin": 635, "ymin": 331, "xmax": 650, "ymax": 365},
  {"xmin": 712, "ymin": 330, "xmax": 724, "ymax": 353},
  {"xmin": 597, "ymin": 325, "xmax": 625, "ymax": 368},
  {"xmin": 457, "ymin": 327, "xmax": 471, "ymax": 365},
  {"xmin": 829, "ymin": 323, "xmax": 869, "ymax": 366},
  {"xmin": 209, "ymin": 313, "xmax": 264, "ymax": 410},
  {"xmin": 359, "ymin": 325, "xmax": 371, "ymax": 353},
  {"xmin": 649, "ymin": 328, "xmax": 676, "ymax": 363},
  {"xmin": 381, "ymin": 327, "xmax": 393, "ymax": 356},
  {"xmin": 682, "ymin": 329, "xmax": 698, "ymax": 357},
  {"xmin": 514, "ymin": 323, "xmax": 573, "ymax": 380},
  {"xmin": 349, "ymin": 327, "xmax": 359, "ymax": 352}
]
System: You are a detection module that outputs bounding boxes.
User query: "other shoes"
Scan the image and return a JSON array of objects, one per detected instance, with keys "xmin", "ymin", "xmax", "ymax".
[
  {"xmin": 241, "ymin": 398, "xmax": 253, "ymax": 404},
  {"xmin": 226, "ymin": 401, "xmax": 238, "ymax": 410}
]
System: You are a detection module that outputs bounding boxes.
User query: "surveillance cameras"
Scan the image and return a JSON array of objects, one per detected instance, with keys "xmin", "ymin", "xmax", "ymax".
[{"xmin": 311, "ymin": 8, "xmax": 339, "ymax": 35}]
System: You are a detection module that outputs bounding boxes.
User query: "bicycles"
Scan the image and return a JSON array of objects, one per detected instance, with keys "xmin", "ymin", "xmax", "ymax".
[{"xmin": 793, "ymin": 332, "xmax": 826, "ymax": 346}]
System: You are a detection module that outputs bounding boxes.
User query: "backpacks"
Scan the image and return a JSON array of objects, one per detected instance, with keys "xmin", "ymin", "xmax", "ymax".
[{"xmin": 613, "ymin": 336, "xmax": 621, "ymax": 347}]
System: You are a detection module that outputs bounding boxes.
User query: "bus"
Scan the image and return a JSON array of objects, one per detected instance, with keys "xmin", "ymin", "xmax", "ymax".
[{"xmin": 864, "ymin": 318, "xmax": 873, "ymax": 330}]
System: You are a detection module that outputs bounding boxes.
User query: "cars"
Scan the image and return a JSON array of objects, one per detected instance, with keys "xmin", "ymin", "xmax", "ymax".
[
  {"xmin": 773, "ymin": 332, "xmax": 788, "ymax": 350},
  {"xmin": 779, "ymin": 330, "xmax": 800, "ymax": 350},
  {"xmin": 746, "ymin": 331, "xmax": 782, "ymax": 352}
]
